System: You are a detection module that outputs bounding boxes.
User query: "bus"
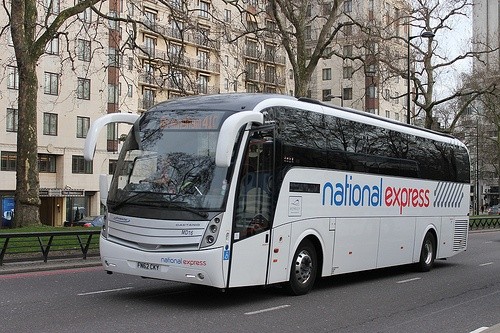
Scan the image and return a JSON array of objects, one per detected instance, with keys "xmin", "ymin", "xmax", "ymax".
[{"xmin": 83, "ymin": 92, "xmax": 470, "ymax": 296}]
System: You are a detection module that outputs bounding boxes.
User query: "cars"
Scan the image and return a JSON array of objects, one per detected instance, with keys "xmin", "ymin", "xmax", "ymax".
[
  {"xmin": 488, "ymin": 204, "xmax": 500, "ymax": 215},
  {"xmin": 77, "ymin": 216, "xmax": 104, "ymax": 227}
]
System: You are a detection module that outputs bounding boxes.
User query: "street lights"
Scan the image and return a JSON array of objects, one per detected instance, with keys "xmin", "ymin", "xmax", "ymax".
[{"xmin": 407, "ymin": 31, "xmax": 435, "ymax": 125}]
